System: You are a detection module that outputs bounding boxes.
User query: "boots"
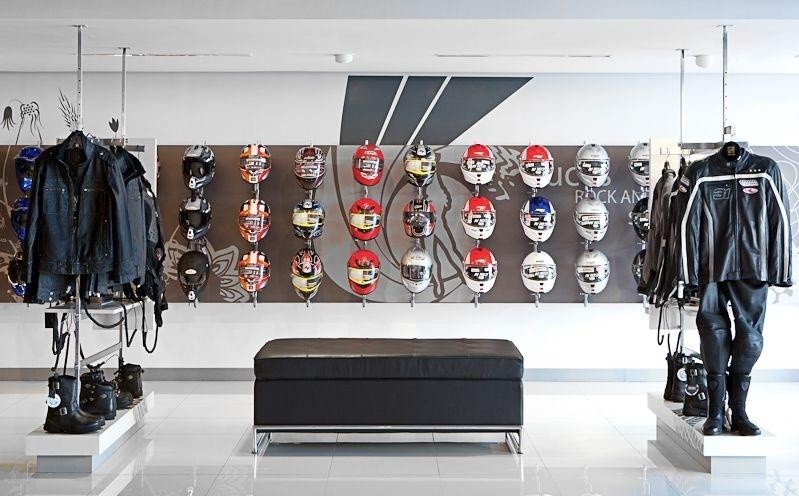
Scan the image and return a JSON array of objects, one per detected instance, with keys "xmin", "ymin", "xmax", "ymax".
[
  {"xmin": 115, "ymin": 363, "xmax": 143, "ymax": 398},
  {"xmin": 700, "ymin": 369, "xmax": 727, "ymax": 435},
  {"xmin": 79, "ymin": 370, "xmax": 119, "ymax": 421},
  {"xmin": 661, "ymin": 348, "xmax": 688, "ymax": 401},
  {"xmin": 728, "ymin": 372, "xmax": 761, "ymax": 436},
  {"xmin": 42, "ymin": 372, "xmax": 106, "ymax": 435},
  {"xmin": 679, "ymin": 352, "xmax": 708, "ymax": 416}
]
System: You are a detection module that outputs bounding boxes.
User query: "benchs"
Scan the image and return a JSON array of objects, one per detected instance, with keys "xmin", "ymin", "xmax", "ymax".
[{"xmin": 252, "ymin": 338, "xmax": 524, "ymax": 455}]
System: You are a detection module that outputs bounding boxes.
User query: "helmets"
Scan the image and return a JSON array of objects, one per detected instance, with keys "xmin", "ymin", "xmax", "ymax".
[
  {"xmin": 346, "ymin": 249, "xmax": 382, "ymax": 297},
  {"xmin": 288, "ymin": 197, "xmax": 326, "ymax": 241},
  {"xmin": 630, "ymin": 248, "xmax": 646, "ymax": 285},
  {"xmin": 572, "ymin": 195, "xmax": 610, "ymax": 243},
  {"xmin": 460, "ymin": 195, "xmax": 498, "ymax": 240},
  {"xmin": 237, "ymin": 144, "xmax": 273, "ymax": 185},
  {"xmin": 176, "ymin": 195, "xmax": 213, "ymax": 240},
  {"xmin": 462, "ymin": 247, "xmax": 499, "ymax": 295},
  {"xmin": 351, "ymin": 144, "xmax": 385, "ymax": 188},
  {"xmin": 292, "ymin": 145, "xmax": 328, "ymax": 192},
  {"xmin": 573, "ymin": 144, "xmax": 612, "ymax": 188},
  {"xmin": 631, "ymin": 196, "xmax": 648, "ymax": 243},
  {"xmin": 400, "ymin": 195, "xmax": 438, "ymax": 239},
  {"xmin": 6, "ymin": 144, "xmax": 46, "ymax": 299},
  {"xmin": 399, "ymin": 246, "xmax": 434, "ymax": 295},
  {"xmin": 289, "ymin": 248, "xmax": 325, "ymax": 299},
  {"xmin": 345, "ymin": 198, "xmax": 384, "ymax": 242},
  {"xmin": 402, "ymin": 144, "xmax": 438, "ymax": 189},
  {"xmin": 181, "ymin": 144, "xmax": 216, "ymax": 193},
  {"xmin": 518, "ymin": 195, "xmax": 557, "ymax": 244},
  {"xmin": 235, "ymin": 197, "xmax": 273, "ymax": 243},
  {"xmin": 519, "ymin": 249, "xmax": 558, "ymax": 293},
  {"xmin": 175, "ymin": 249, "xmax": 211, "ymax": 301},
  {"xmin": 574, "ymin": 249, "xmax": 611, "ymax": 295},
  {"xmin": 517, "ymin": 145, "xmax": 555, "ymax": 190},
  {"xmin": 627, "ymin": 142, "xmax": 650, "ymax": 188},
  {"xmin": 236, "ymin": 249, "xmax": 273, "ymax": 294},
  {"xmin": 460, "ymin": 143, "xmax": 496, "ymax": 185}
]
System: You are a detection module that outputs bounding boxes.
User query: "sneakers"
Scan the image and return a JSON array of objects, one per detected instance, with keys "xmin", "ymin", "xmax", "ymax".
[{"xmin": 110, "ymin": 378, "xmax": 133, "ymax": 410}]
